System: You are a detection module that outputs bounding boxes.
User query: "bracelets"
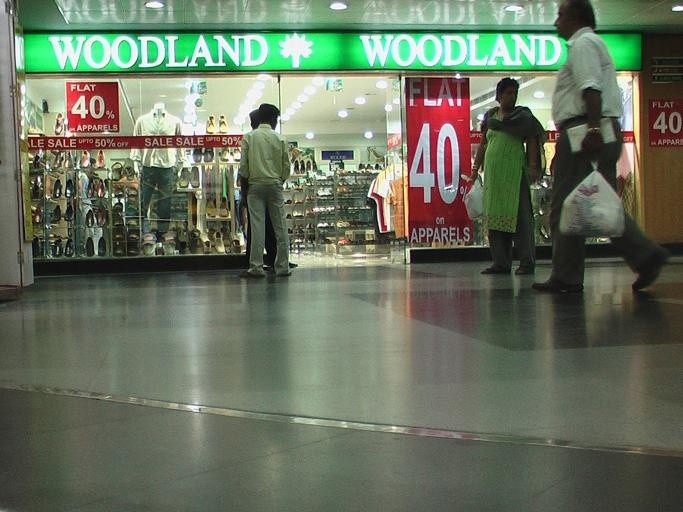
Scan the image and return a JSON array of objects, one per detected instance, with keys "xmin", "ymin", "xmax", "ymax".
[
  {"xmin": 587, "ymin": 127, "xmax": 599, "ymax": 132},
  {"xmin": 472, "ymin": 165, "xmax": 479, "ymax": 170},
  {"xmin": 529, "ymin": 160, "xmax": 538, "ymax": 169}
]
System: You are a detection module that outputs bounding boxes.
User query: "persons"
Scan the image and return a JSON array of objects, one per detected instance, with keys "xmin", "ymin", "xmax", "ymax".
[
  {"xmin": 529, "ymin": 0, "xmax": 670, "ymax": 294},
  {"xmin": 77, "ymin": 158, "xmax": 98, "ymax": 254},
  {"xmin": 130, "ymin": 101, "xmax": 187, "ymax": 256},
  {"xmin": 239, "ymin": 103, "xmax": 293, "ymax": 277},
  {"xmin": 238, "ymin": 110, "xmax": 277, "ymax": 273},
  {"xmin": 466, "ymin": 76, "xmax": 544, "ymax": 275}
]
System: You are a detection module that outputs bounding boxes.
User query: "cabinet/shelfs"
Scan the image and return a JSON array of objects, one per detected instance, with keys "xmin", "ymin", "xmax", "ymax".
[{"xmin": 283, "ymin": 170, "xmax": 390, "ymax": 255}]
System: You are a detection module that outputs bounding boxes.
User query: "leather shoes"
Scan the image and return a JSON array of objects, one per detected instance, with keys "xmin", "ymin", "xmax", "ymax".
[
  {"xmin": 206, "ymin": 115, "xmax": 215, "ymax": 132},
  {"xmin": 218, "ymin": 114, "xmax": 228, "ymax": 133},
  {"xmin": 54, "ymin": 112, "xmax": 65, "ymax": 135}
]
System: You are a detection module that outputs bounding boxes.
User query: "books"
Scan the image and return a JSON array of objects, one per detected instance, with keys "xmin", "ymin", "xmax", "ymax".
[{"xmin": 566, "ymin": 117, "xmax": 619, "ymax": 153}]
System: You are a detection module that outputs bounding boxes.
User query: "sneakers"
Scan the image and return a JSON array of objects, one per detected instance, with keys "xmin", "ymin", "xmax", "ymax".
[
  {"xmin": 30, "ymin": 146, "xmax": 243, "ymax": 256},
  {"xmin": 480, "ymin": 264, "xmax": 511, "ymax": 274},
  {"xmin": 531, "ymin": 278, "xmax": 584, "ymax": 297},
  {"xmin": 631, "ymin": 246, "xmax": 669, "ymax": 290},
  {"xmin": 515, "ymin": 266, "xmax": 535, "ymax": 274},
  {"xmin": 282, "ymin": 145, "xmax": 385, "ymax": 247}
]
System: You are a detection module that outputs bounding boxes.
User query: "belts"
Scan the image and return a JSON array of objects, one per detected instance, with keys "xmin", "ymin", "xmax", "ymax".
[{"xmin": 558, "ymin": 114, "xmax": 590, "ymax": 130}]
